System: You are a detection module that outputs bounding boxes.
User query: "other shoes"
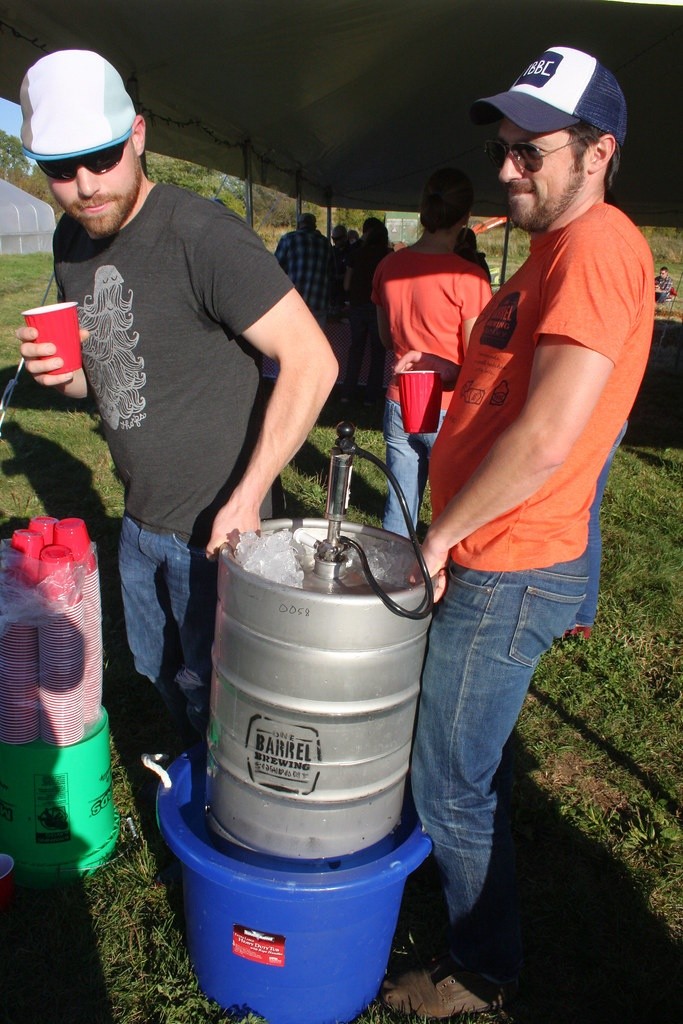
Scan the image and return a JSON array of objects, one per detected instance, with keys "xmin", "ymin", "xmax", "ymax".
[
  {"xmin": 417, "ymin": 854, "xmax": 441, "ymax": 880},
  {"xmin": 341, "ymin": 392, "xmax": 357, "ymax": 403},
  {"xmin": 380, "ymin": 950, "xmax": 518, "ymax": 1019},
  {"xmin": 363, "ymin": 394, "xmax": 377, "ymax": 406}
]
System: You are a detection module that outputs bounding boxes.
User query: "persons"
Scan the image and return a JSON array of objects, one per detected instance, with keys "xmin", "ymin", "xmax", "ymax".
[
  {"xmin": 370, "ymin": 168, "xmax": 493, "ymax": 539},
  {"xmin": 340, "ymin": 218, "xmax": 394, "ymax": 406},
  {"xmin": 347, "ymin": 230, "xmax": 359, "ymax": 246},
  {"xmin": 378, "ymin": 48, "xmax": 655, "ymax": 1019},
  {"xmin": 655, "ymin": 267, "xmax": 673, "ymax": 303},
  {"xmin": 329, "ymin": 225, "xmax": 353, "ymax": 313},
  {"xmin": 275, "ymin": 212, "xmax": 336, "ymax": 329},
  {"xmin": 16, "ymin": 50, "xmax": 340, "ymax": 804},
  {"xmin": 455, "ymin": 228, "xmax": 491, "ymax": 283}
]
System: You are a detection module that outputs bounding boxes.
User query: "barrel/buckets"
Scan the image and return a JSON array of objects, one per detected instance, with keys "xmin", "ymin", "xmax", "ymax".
[
  {"xmin": 0, "ymin": 705, "xmax": 120, "ymax": 890},
  {"xmin": 208, "ymin": 519, "xmax": 433, "ymax": 859},
  {"xmin": 155, "ymin": 741, "xmax": 434, "ymax": 1024}
]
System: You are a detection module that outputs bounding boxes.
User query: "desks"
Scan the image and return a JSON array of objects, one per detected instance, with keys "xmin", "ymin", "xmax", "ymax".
[{"xmin": 259, "ymin": 319, "xmax": 394, "ymax": 390}]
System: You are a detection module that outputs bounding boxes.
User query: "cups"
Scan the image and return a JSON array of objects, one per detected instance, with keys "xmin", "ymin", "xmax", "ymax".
[
  {"xmin": 396, "ymin": 370, "xmax": 441, "ymax": 434},
  {"xmin": 0, "ymin": 854, "xmax": 14, "ymax": 895},
  {"xmin": 20, "ymin": 302, "xmax": 82, "ymax": 375},
  {"xmin": 0, "ymin": 517, "xmax": 102, "ymax": 747}
]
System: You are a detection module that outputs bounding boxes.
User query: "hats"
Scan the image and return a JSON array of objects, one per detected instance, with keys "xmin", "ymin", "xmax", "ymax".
[
  {"xmin": 334, "ymin": 225, "xmax": 347, "ymax": 232},
  {"xmin": 472, "ymin": 47, "xmax": 627, "ymax": 149},
  {"xmin": 19, "ymin": 49, "xmax": 137, "ymax": 160},
  {"xmin": 298, "ymin": 213, "xmax": 316, "ymax": 223}
]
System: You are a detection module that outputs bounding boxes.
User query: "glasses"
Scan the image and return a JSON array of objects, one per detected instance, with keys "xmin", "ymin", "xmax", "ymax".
[
  {"xmin": 483, "ymin": 138, "xmax": 582, "ymax": 173},
  {"xmin": 36, "ymin": 141, "xmax": 125, "ymax": 181},
  {"xmin": 332, "ymin": 234, "xmax": 345, "ymax": 241}
]
